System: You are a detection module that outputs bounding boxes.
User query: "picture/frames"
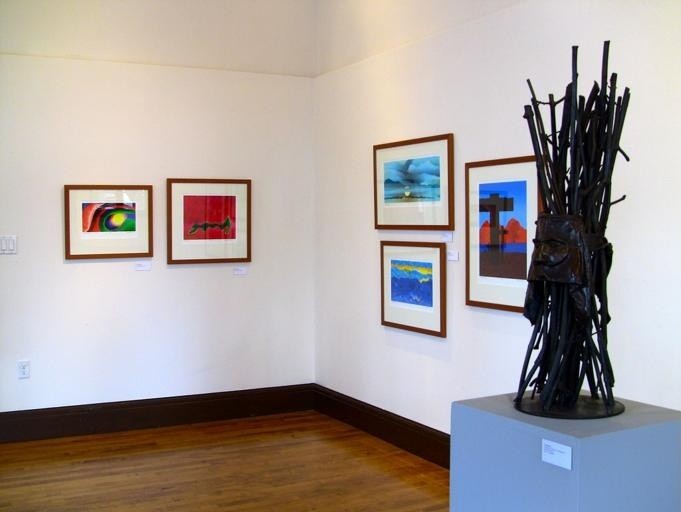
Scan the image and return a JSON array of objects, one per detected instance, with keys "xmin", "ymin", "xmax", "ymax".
[
  {"xmin": 63, "ymin": 184, "xmax": 153, "ymax": 260},
  {"xmin": 465, "ymin": 154, "xmax": 544, "ymax": 312},
  {"xmin": 380, "ymin": 240, "xmax": 447, "ymax": 339},
  {"xmin": 372, "ymin": 133, "xmax": 455, "ymax": 231},
  {"xmin": 167, "ymin": 177, "xmax": 252, "ymax": 265}
]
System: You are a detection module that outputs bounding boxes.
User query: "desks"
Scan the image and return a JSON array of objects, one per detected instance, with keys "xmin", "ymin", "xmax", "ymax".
[{"xmin": 449, "ymin": 389, "xmax": 681, "ymax": 511}]
